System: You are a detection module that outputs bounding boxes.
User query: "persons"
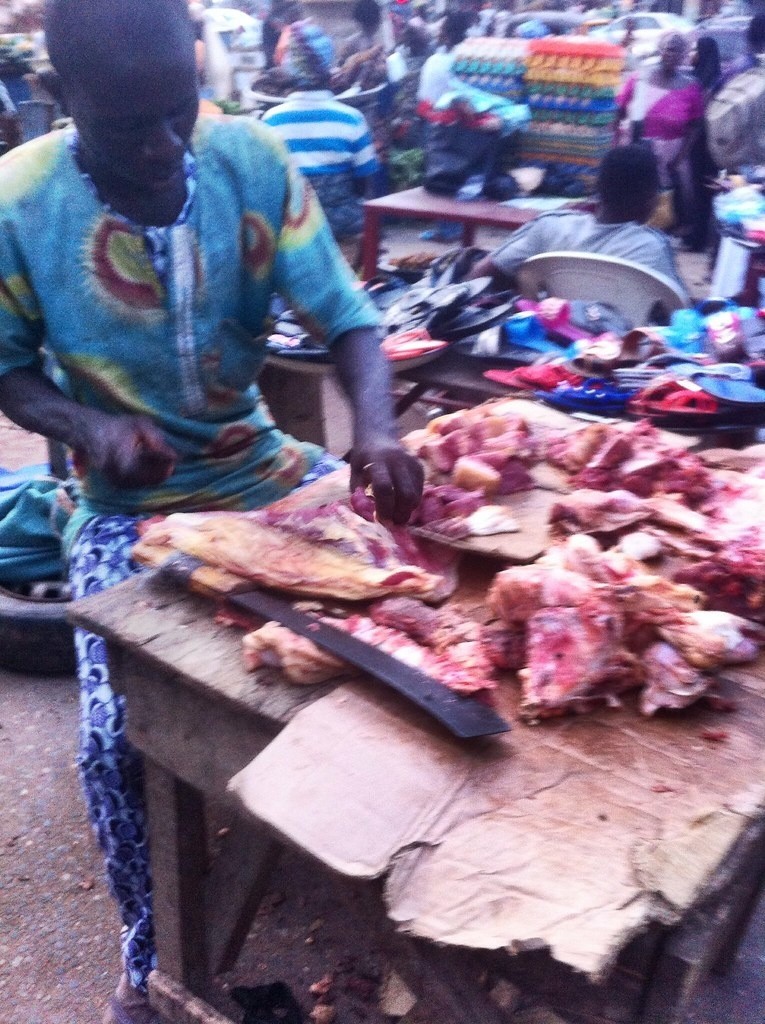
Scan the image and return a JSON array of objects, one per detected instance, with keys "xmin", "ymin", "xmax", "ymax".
[
  {"xmin": 461, "ymin": 143, "xmax": 682, "ymax": 288},
  {"xmin": 0, "ymin": 1, "xmax": 429, "ymax": 1023},
  {"xmin": 263, "ymin": 50, "xmax": 382, "ymax": 239},
  {"xmin": 190, "ymin": 0, "xmax": 765, "ymax": 252}
]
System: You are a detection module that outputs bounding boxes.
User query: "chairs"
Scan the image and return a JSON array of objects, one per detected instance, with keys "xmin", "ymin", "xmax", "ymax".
[{"xmin": 514, "ymin": 249, "xmax": 697, "ymax": 329}]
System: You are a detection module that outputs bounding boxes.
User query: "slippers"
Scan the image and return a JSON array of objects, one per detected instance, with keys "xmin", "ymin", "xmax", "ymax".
[
  {"xmin": 265, "ymin": 263, "xmax": 765, "ymax": 425},
  {"xmin": 103, "ymin": 994, "xmax": 150, "ymax": 1024}
]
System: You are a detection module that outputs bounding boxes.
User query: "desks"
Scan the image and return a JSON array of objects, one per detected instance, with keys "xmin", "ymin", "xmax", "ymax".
[
  {"xmin": 61, "ymin": 346, "xmax": 765, "ymax": 1024},
  {"xmin": 359, "ymin": 185, "xmax": 543, "ymax": 282}
]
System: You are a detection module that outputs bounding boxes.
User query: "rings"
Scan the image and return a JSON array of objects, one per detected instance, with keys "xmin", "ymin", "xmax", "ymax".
[{"xmin": 363, "ymin": 462, "xmax": 375, "ymax": 470}]
